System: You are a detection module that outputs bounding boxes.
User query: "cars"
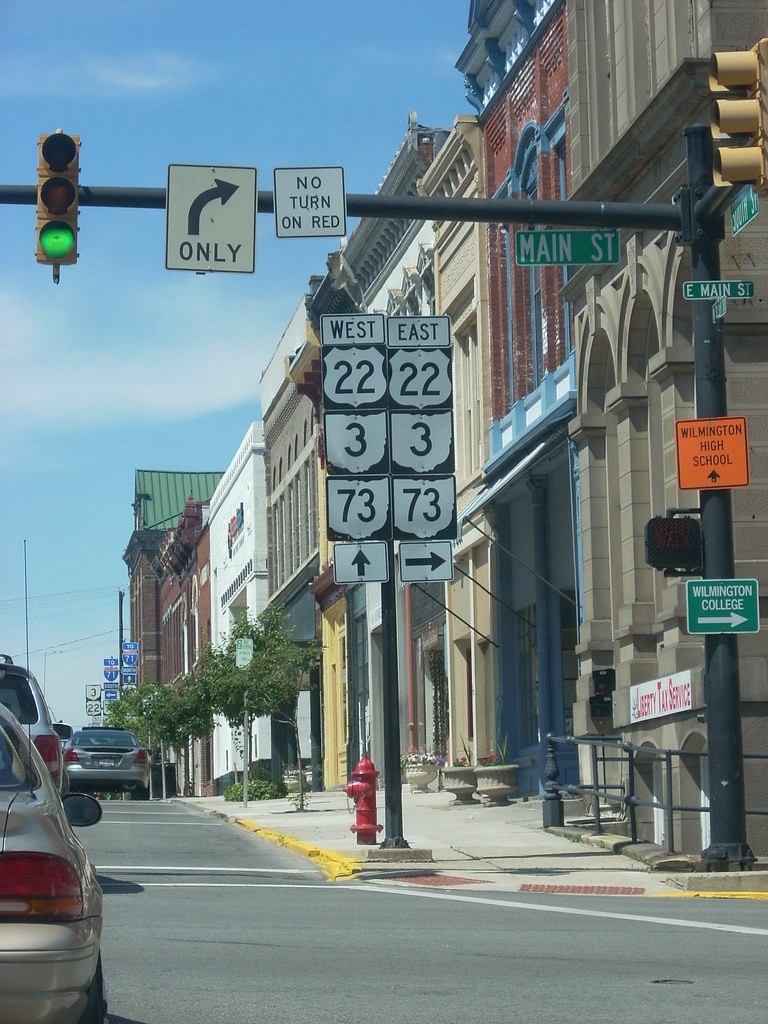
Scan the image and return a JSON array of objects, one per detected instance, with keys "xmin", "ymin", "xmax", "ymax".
[
  {"xmin": 63, "ymin": 729, "xmax": 151, "ymax": 802},
  {"xmin": 0, "ymin": 704, "xmax": 105, "ymax": 1024},
  {"xmin": 0, "ymin": 654, "xmax": 73, "ymax": 797},
  {"xmin": 52, "ymin": 722, "xmax": 73, "ymax": 750}
]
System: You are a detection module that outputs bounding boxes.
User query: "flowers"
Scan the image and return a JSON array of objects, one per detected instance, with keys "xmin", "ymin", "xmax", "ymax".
[
  {"xmin": 477, "ymin": 736, "xmax": 509, "ymax": 766},
  {"xmin": 400, "ymin": 753, "xmax": 444, "ymax": 766},
  {"xmin": 450, "ymin": 757, "xmax": 468, "ymax": 766},
  {"xmin": 280, "ymin": 758, "xmax": 313, "ymax": 770}
]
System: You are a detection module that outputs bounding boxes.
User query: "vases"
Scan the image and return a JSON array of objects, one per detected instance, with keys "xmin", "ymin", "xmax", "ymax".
[
  {"xmin": 406, "ymin": 766, "xmax": 439, "ymax": 794},
  {"xmin": 473, "ymin": 765, "xmax": 519, "ymax": 807},
  {"xmin": 441, "ymin": 766, "xmax": 475, "ymax": 805},
  {"xmin": 283, "ymin": 769, "xmax": 314, "ymax": 792}
]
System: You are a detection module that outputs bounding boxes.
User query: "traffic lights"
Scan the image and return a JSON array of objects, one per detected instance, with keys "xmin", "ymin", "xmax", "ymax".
[
  {"xmin": 644, "ymin": 515, "xmax": 702, "ymax": 570},
  {"xmin": 709, "ymin": 38, "xmax": 768, "ymax": 194},
  {"xmin": 36, "ymin": 133, "xmax": 81, "ymax": 265}
]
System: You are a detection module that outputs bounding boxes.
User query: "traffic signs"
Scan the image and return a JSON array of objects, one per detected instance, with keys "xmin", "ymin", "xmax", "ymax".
[
  {"xmin": 730, "ymin": 184, "xmax": 760, "ymax": 237},
  {"xmin": 676, "ymin": 416, "xmax": 751, "ymax": 491},
  {"xmin": 165, "ymin": 164, "xmax": 257, "ymax": 273},
  {"xmin": 515, "ymin": 229, "xmax": 620, "ymax": 267},
  {"xmin": 684, "ymin": 281, "xmax": 754, "ymax": 300},
  {"xmin": 686, "ymin": 579, "xmax": 760, "ymax": 634},
  {"xmin": 712, "ymin": 295, "xmax": 727, "ymax": 323}
]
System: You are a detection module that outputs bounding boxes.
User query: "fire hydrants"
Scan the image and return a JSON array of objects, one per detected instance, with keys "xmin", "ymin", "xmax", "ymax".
[{"xmin": 345, "ymin": 756, "xmax": 383, "ymax": 843}]
